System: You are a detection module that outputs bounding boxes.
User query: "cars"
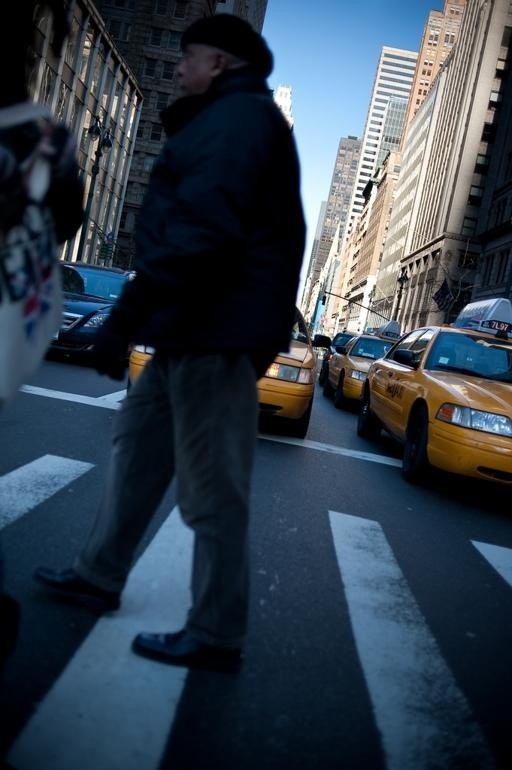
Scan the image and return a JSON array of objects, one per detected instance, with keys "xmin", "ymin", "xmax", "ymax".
[{"xmin": 357, "ymin": 296, "xmax": 512, "ymax": 491}]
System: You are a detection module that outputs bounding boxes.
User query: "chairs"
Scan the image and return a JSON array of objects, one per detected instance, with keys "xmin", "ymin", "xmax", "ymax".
[{"xmin": 435, "ymin": 348, "xmax": 455, "ymax": 368}]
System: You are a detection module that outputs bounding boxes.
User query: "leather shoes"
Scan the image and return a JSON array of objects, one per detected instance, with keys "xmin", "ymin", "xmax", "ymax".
[
  {"xmin": 35, "ymin": 560, "xmax": 119, "ymax": 612},
  {"xmin": 132, "ymin": 627, "xmax": 237, "ymax": 672}
]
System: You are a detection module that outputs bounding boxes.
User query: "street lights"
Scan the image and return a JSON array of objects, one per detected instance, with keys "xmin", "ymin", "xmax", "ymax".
[{"xmin": 74, "ymin": 113, "xmax": 114, "ymax": 262}]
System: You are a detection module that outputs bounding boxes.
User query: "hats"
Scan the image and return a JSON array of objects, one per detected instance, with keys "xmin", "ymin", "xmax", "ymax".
[{"xmin": 179, "ymin": 12, "xmax": 273, "ymax": 79}]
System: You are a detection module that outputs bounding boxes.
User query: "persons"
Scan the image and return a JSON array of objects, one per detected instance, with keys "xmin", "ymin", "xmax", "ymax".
[
  {"xmin": 0, "ymin": 1, "xmax": 90, "ymax": 682},
  {"xmin": 26, "ymin": 14, "xmax": 306, "ymax": 673}
]
